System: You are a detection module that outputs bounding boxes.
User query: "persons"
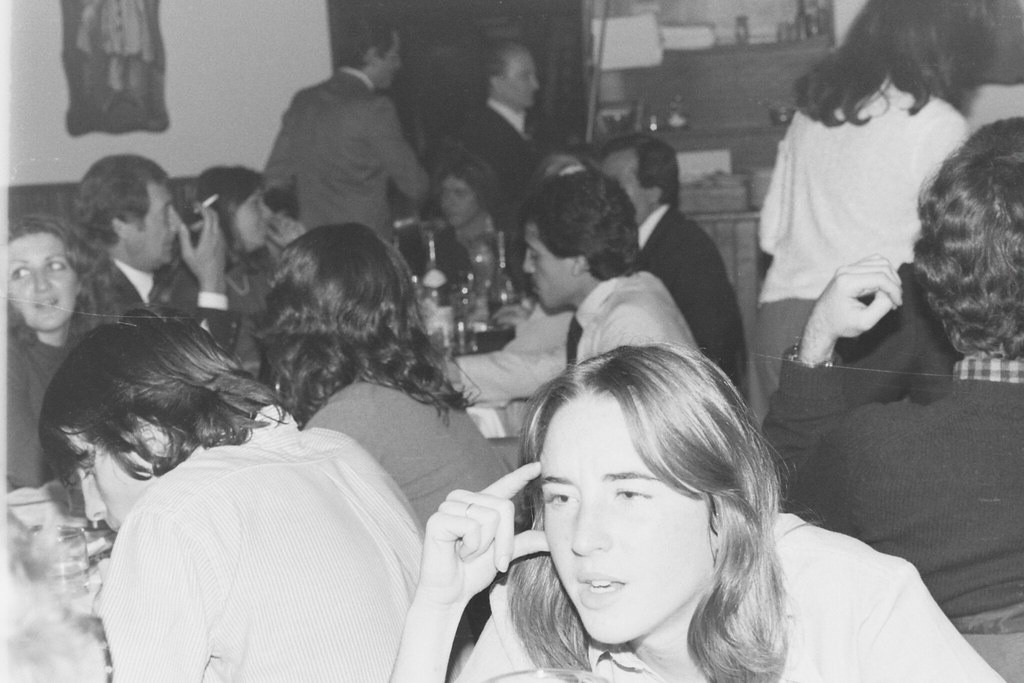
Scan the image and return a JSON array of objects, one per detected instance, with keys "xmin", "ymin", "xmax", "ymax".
[{"xmin": 9, "ymin": 0, "xmax": 1023, "ymax": 683}]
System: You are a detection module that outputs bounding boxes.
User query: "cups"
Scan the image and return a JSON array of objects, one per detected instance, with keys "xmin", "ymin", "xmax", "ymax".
[{"xmin": 33, "ymin": 526, "xmax": 90, "ymax": 599}]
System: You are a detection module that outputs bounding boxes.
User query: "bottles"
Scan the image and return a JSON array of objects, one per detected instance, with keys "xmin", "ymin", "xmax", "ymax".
[
  {"xmin": 491, "ymin": 232, "xmax": 522, "ymax": 331},
  {"xmin": 417, "ymin": 229, "xmax": 457, "ymax": 355}
]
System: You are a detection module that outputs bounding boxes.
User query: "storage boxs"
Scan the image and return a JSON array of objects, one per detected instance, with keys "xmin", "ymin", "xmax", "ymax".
[
  {"xmin": 680, "ymin": 183, "xmax": 749, "ymax": 214},
  {"xmin": 588, "ymin": 13, "xmax": 663, "ymax": 70}
]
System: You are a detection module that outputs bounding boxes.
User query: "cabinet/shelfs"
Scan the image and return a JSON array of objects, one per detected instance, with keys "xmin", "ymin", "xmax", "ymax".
[{"xmin": 582, "ymin": 0, "xmax": 837, "ymax": 432}]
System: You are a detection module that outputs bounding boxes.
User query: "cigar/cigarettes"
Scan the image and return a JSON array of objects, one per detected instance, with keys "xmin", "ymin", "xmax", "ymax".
[{"xmin": 193, "ymin": 193, "xmax": 221, "ymax": 212}]
